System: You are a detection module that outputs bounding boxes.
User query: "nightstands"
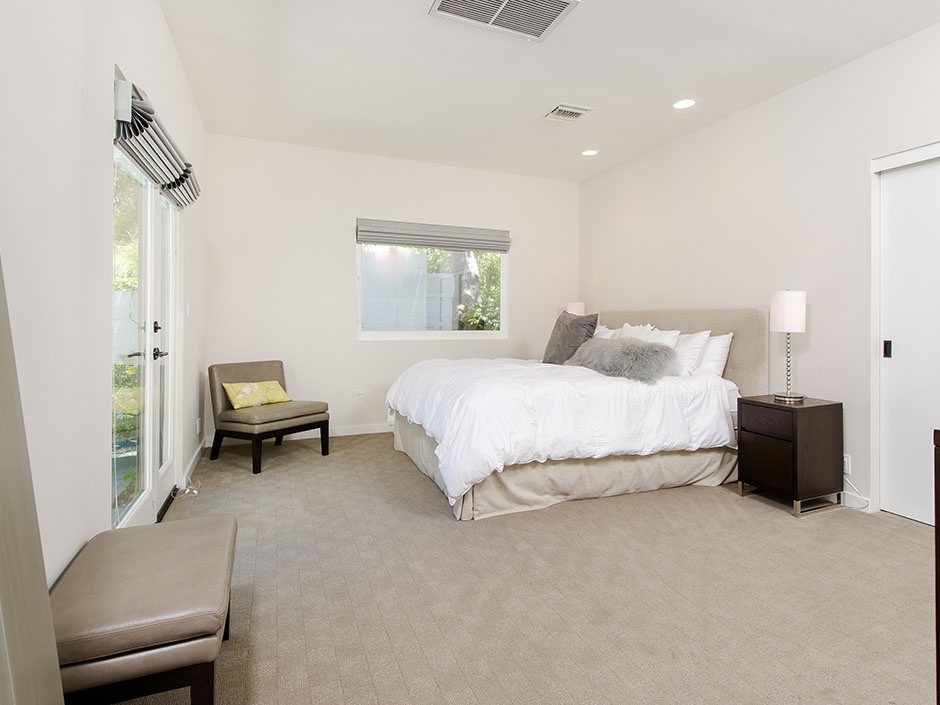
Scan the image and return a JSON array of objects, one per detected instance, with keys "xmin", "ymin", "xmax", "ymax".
[{"xmin": 735, "ymin": 392, "xmax": 845, "ymax": 517}]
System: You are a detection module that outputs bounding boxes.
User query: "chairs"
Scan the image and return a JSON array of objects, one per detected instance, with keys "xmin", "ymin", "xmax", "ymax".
[{"xmin": 206, "ymin": 358, "xmax": 329, "ymax": 475}]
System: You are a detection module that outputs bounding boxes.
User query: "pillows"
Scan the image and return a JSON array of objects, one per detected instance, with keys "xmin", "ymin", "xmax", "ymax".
[
  {"xmin": 542, "ymin": 310, "xmax": 734, "ymax": 384},
  {"xmin": 220, "ymin": 376, "xmax": 292, "ymax": 410}
]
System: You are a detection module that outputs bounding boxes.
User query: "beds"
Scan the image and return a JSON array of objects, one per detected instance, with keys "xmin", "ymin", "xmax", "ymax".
[{"xmin": 385, "ymin": 300, "xmax": 771, "ymax": 525}]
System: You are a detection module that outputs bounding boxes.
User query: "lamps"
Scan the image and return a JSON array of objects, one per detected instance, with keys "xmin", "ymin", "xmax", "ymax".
[
  {"xmin": 769, "ymin": 288, "xmax": 810, "ymax": 403},
  {"xmin": 565, "ymin": 302, "xmax": 585, "ymax": 318}
]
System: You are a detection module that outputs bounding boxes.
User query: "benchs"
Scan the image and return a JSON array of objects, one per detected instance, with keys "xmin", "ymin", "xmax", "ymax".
[{"xmin": 45, "ymin": 515, "xmax": 236, "ymax": 705}]
